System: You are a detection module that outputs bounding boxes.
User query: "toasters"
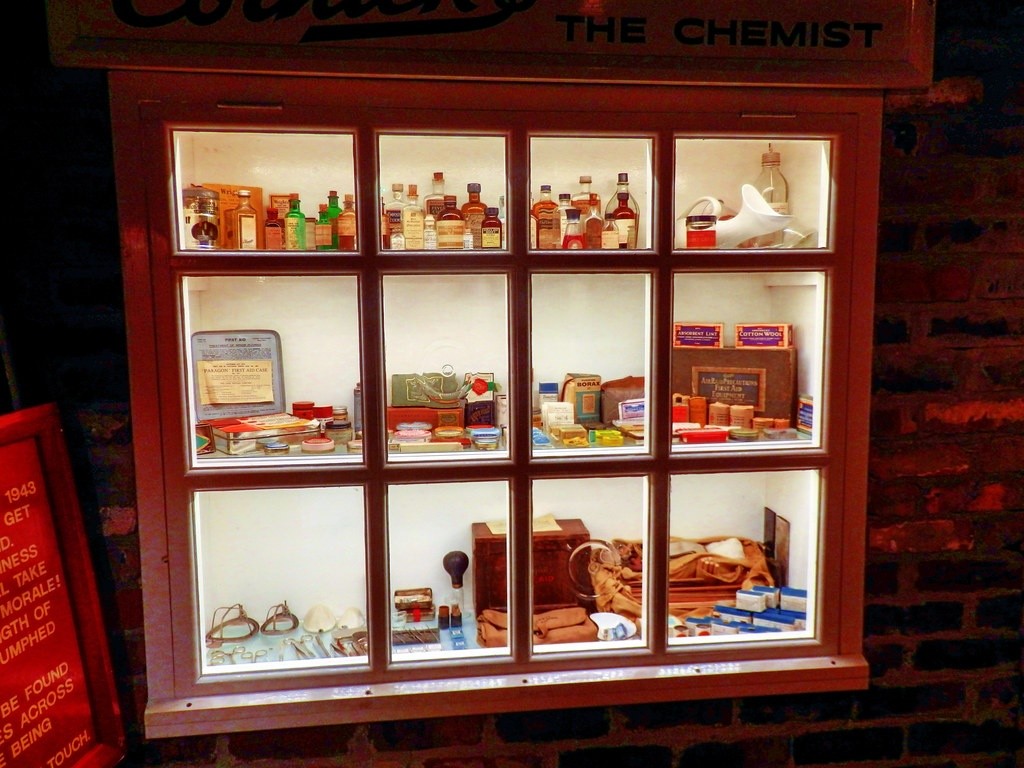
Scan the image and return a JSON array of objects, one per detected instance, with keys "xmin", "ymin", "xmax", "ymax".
[{"xmin": 262, "ymin": 208, "xmax": 282, "ymax": 249}]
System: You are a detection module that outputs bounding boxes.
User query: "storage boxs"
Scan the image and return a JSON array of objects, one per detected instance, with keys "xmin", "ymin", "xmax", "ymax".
[
  {"xmin": 672, "ymin": 345, "xmax": 798, "ymax": 424},
  {"xmin": 734, "ymin": 324, "xmax": 793, "ymax": 349},
  {"xmin": 672, "ymin": 322, "xmax": 723, "ymax": 348},
  {"xmin": 471, "ymin": 519, "xmax": 593, "ymax": 621}
]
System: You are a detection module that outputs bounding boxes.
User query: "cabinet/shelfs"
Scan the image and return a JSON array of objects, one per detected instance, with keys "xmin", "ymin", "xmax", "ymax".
[{"xmin": 146, "ymin": 104, "xmax": 858, "ymax": 693}]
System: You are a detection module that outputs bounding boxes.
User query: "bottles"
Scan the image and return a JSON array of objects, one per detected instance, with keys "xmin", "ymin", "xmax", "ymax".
[
  {"xmin": 530, "ymin": 173, "xmax": 640, "ymax": 249},
  {"xmin": 233, "ymin": 190, "xmax": 258, "ymax": 250},
  {"xmin": 183, "ymin": 187, "xmax": 220, "ymax": 250},
  {"xmin": 382, "ymin": 184, "xmax": 425, "ymax": 249},
  {"xmin": 292, "ymin": 401, "xmax": 353, "ymax": 444},
  {"xmin": 284, "ymin": 194, "xmax": 306, "ymax": 251},
  {"xmin": 718, "ymin": 198, "xmax": 754, "ymax": 248},
  {"xmin": 673, "ymin": 392, "xmax": 790, "ymax": 430},
  {"xmin": 314, "ymin": 190, "xmax": 356, "ymax": 252},
  {"xmin": 538, "ymin": 383, "xmax": 559, "ymax": 408},
  {"xmin": 423, "ymin": 172, "xmax": 465, "ymax": 250},
  {"xmin": 685, "ymin": 214, "xmax": 717, "ymax": 248},
  {"xmin": 754, "ymin": 152, "xmax": 789, "ymax": 248},
  {"xmin": 461, "ymin": 183, "xmax": 506, "ymax": 250}
]
